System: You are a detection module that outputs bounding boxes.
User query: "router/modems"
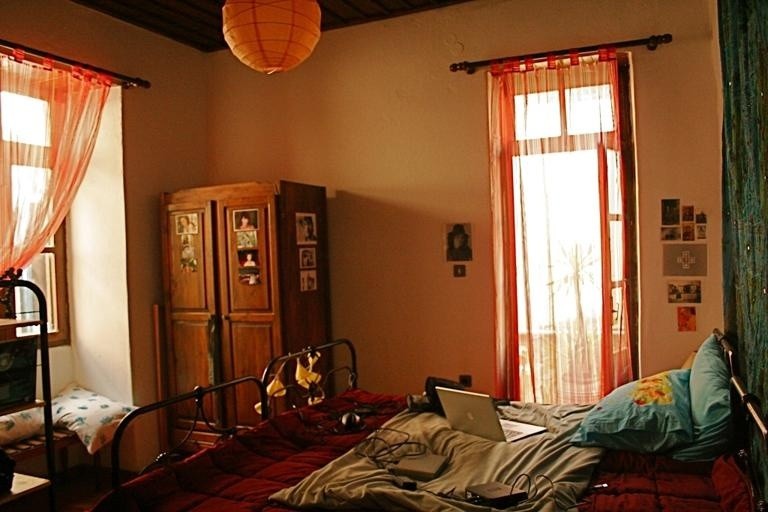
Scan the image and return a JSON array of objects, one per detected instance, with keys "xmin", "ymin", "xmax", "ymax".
[{"xmin": 465, "ymin": 481, "xmax": 529, "ymax": 510}]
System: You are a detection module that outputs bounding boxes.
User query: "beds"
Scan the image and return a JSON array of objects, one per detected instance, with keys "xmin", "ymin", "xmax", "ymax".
[
  {"xmin": 110, "ymin": 327, "xmax": 766, "ymax": 511},
  {"xmin": 0, "ymin": 280, "xmax": 57, "ymax": 511}
]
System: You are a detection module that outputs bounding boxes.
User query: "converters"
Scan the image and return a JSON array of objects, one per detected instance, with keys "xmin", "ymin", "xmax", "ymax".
[{"xmin": 395, "ymin": 475, "xmax": 417, "ymax": 490}]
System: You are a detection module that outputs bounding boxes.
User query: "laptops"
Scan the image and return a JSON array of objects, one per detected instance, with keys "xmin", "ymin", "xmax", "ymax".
[{"xmin": 435, "ymin": 385, "xmax": 549, "ymax": 443}]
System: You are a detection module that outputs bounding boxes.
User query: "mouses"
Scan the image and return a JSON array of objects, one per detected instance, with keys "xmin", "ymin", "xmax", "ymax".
[{"xmin": 342, "ymin": 411, "xmax": 361, "ymax": 426}]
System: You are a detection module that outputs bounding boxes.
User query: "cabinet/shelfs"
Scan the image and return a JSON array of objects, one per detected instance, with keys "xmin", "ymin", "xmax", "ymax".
[{"xmin": 158, "ymin": 178, "xmax": 333, "ymax": 451}]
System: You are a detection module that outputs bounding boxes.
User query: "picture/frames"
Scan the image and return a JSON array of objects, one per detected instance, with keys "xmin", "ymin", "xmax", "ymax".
[{"xmin": 443, "ymin": 219, "xmax": 473, "ymax": 263}]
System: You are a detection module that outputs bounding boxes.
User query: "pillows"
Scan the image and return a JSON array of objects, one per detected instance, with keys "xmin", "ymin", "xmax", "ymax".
[
  {"xmin": 1, "ymin": 385, "xmax": 140, "ymax": 455},
  {"xmin": 569, "ymin": 367, "xmax": 694, "ymax": 453},
  {"xmin": 673, "ymin": 332, "xmax": 736, "ymax": 473}
]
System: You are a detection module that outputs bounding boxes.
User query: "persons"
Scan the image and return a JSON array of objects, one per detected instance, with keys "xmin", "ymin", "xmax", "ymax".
[
  {"xmin": 447, "ymin": 224, "xmax": 472, "ymax": 262},
  {"xmin": 177, "ymin": 216, "xmax": 199, "ymax": 233},
  {"xmin": 241, "ymin": 252, "xmax": 255, "ymax": 267},
  {"xmin": 299, "ymin": 215, "xmax": 315, "ymax": 240}
]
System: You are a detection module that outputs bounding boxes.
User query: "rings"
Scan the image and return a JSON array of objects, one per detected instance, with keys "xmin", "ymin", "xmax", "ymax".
[{"xmin": 237, "ymin": 212, "xmax": 257, "ymax": 229}]
